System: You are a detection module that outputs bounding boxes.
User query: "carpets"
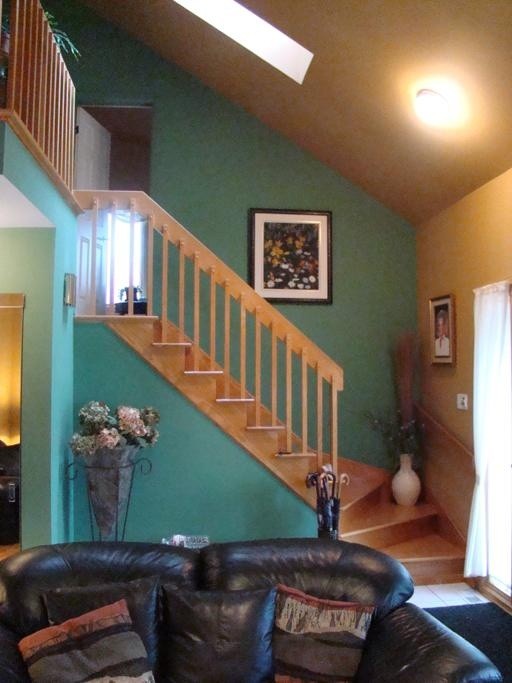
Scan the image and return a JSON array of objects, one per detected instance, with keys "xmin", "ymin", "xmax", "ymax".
[{"xmin": 423, "ymin": 601, "xmax": 512, "ymax": 681}]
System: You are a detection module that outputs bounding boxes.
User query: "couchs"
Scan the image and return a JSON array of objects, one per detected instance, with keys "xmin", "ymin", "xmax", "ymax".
[{"xmin": 0, "ymin": 537, "xmax": 504, "ymax": 682}]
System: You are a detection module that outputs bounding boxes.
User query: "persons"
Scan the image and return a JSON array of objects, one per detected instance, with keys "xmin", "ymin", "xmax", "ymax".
[{"xmin": 435, "ymin": 310, "xmax": 449, "ymax": 358}]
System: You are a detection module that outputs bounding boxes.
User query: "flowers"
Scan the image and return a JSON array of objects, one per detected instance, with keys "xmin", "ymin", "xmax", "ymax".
[
  {"xmin": 67, "ymin": 401, "xmax": 161, "ymax": 460},
  {"xmin": 366, "ymin": 410, "xmax": 425, "ymax": 455}
]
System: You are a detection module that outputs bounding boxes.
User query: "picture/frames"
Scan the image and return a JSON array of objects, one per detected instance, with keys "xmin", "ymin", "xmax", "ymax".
[
  {"xmin": 248, "ymin": 207, "xmax": 334, "ymax": 306},
  {"xmin": 427, "ymin": 293, "xmax": 458, "ymax": 364}
]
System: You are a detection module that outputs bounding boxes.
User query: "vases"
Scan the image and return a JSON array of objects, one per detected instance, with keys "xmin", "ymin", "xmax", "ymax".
[
  {"xmin": 83, "ymin": 444, "xmax": 140, "ymax": 538},
  {"xmin": 391, "ymin": 452, "xmax": 422, "ymax": 505}
]
